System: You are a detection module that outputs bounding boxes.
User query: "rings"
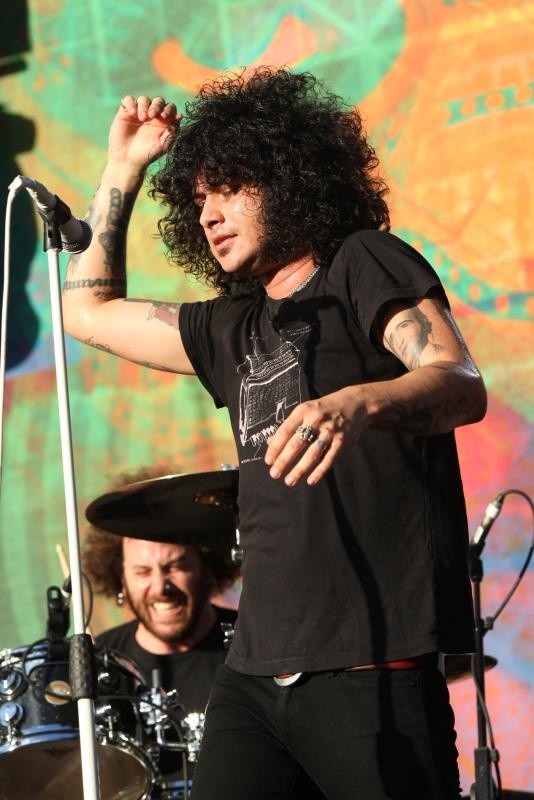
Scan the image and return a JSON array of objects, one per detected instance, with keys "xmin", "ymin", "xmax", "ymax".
[
  {"xmin": 120, "ymin": 99, "xmax": 127, "ymax": 109},
  {"xmin": 295, "ymin": 424, "xmax": 317, "ymax": 442}
]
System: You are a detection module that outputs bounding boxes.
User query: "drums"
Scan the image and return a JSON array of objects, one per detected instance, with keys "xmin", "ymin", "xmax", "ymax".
[{"xmin": 0, "ymin": 638, "xmax": 170, "ymax": 800}]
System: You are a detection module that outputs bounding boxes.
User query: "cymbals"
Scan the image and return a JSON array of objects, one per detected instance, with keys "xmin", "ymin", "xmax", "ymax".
[{"xmin": 84, "ymin": 470, "xmax": 239, "ymax": 545}]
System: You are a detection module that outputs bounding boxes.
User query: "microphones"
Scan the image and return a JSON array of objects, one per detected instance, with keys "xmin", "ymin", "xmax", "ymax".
[
  {"xmin": 470, "ymin": 491, "xmax": 505, "ymax": 557},
  {"xmin": 50, "ymin": 575, "xmax": 72, "ymax": 638},
  {"xmin": 20, "ymin": 176, "xmax": 94, "ymax": 254}
]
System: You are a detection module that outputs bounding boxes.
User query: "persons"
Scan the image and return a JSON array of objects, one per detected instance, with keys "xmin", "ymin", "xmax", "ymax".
[
  {"xmin": 75, "ymin": 460, "xmax": 239, "ymax": 800},
  {"xmin": 60, "ymin": 66, "xmax": 488, "ymax": 800}
]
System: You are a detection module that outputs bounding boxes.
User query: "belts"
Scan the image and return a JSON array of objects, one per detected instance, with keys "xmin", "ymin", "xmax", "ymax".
[{"xmin": 270, "ymin": 652, "xmax": 429, "ymax": 689}]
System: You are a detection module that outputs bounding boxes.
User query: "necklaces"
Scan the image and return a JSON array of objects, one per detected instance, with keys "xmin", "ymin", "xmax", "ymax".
[{"xmin": 286, "ymin": 265, "xmax": 321, "ymax": 298}]
